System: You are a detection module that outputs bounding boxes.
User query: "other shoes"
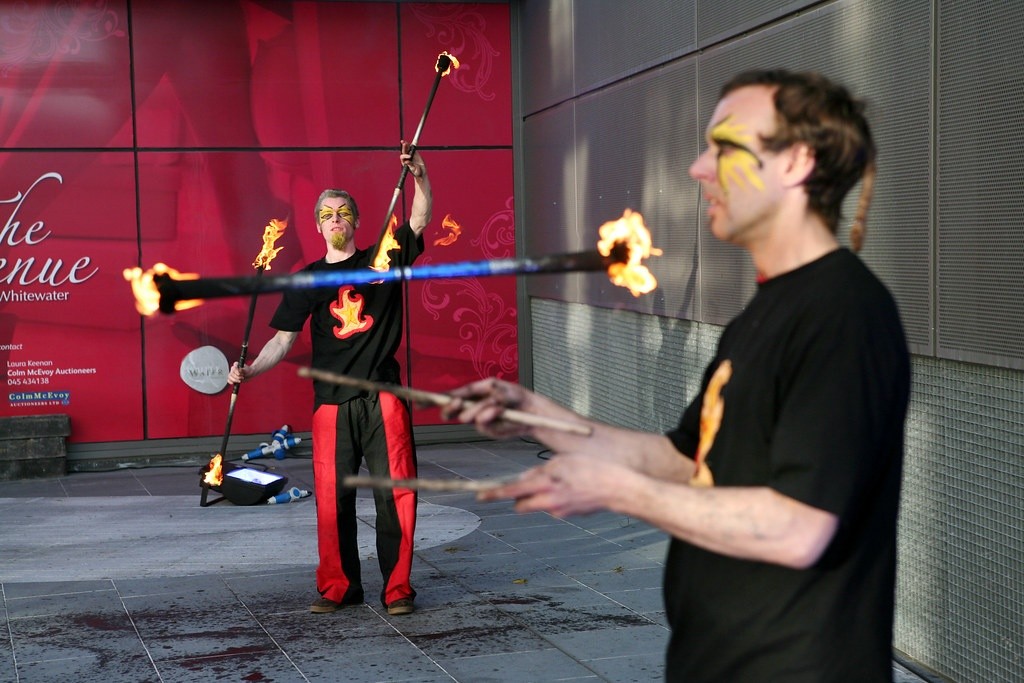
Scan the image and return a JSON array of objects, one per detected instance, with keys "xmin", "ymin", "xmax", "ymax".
[
  {"xmin": 387, "ymin": 598, "xmax": 414, "ymax": 615},
  {"xmin": 309, "ymin": 594, "xmax": 365, "ymax": 613}
]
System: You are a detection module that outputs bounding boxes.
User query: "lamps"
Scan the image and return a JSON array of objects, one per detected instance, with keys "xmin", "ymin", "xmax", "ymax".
[{"xmin": 197, "ymin": 458, "xmax": 286, "ymax": 507}]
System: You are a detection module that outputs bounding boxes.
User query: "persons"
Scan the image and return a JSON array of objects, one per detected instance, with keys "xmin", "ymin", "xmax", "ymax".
[
  {"xmin": 443, "ymin": 68, "xmax": 913, "ymax": 683},
  {"xmin": 226, "ymin": 139, "xmax": 432, "ymax": 613}
]
